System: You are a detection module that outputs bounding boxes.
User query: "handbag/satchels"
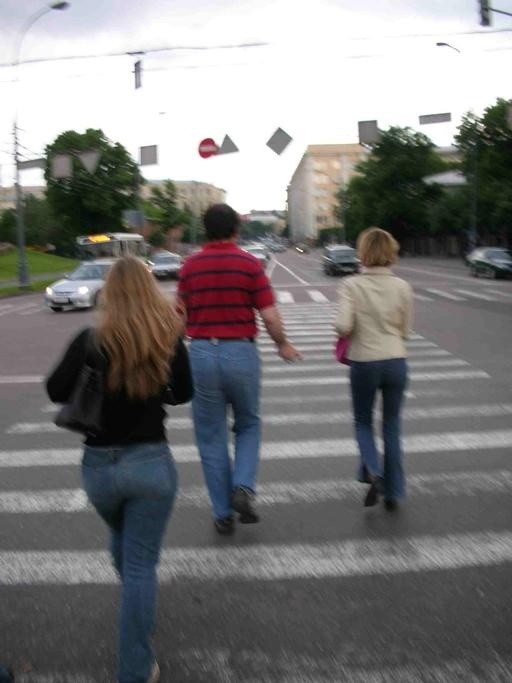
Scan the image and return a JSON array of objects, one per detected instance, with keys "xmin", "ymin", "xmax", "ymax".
[{"xmin": 52, "ymin": 326, "xmax": 109, "ymax": 441}]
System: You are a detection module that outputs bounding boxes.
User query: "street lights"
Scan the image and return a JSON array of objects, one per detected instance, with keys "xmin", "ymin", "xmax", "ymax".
[
  {"xmin": 6, "ymin": 0, "xmax": 71, "ymax": 290},
  {"xmin": 434, "ymin": 35, "xmax": 473, "ymax": 262}
]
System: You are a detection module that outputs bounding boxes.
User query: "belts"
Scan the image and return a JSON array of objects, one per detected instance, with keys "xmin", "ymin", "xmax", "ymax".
[{"xmin": 189, "ymin": 336, "xmax": 254, "ymax": 342}]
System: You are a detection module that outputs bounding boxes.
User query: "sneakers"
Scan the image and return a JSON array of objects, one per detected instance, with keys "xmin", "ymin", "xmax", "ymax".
[
  {"xmin": 364, "ymin": 477, "xmax": 399, "ymax": 513},
  {"xmin": 148, "ymin": 659, "xmax": 161, "ymax": 683},
  {"xmin": 230, "ymin": 490, "xmax": 258, "ymax": 524},
  {"xmin": 214, "ymin": 514, "xmax": 234, "ymax": 533}
]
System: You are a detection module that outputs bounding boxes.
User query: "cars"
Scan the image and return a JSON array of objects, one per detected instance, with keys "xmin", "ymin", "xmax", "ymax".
[
  {"xmin": 462, "ymin": 244, "xmax": 511, "ymax": 281},
  {"xmin": 150, "ymin": 252, "xmax": 183, "ymax": 280},
  {"xmin": 320, "ymin": 242, "xmax": 362, "ymax": 277},
  {"xmin": 43, "ymin": 254, "xmax": 121, "ymax": 314}
]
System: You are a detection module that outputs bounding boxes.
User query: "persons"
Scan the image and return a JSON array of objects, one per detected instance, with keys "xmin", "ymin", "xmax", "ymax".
[
  {"xmin": 174, "ymin": 201, "xmax": 301, "ymax": 536},
  {"xmin": 43, "ymin": 255, "xmax": 198, "ymax": 681},
  {"xmin": 327, "ymin": 224, "xmax": 417, "ymax": 515}
]
